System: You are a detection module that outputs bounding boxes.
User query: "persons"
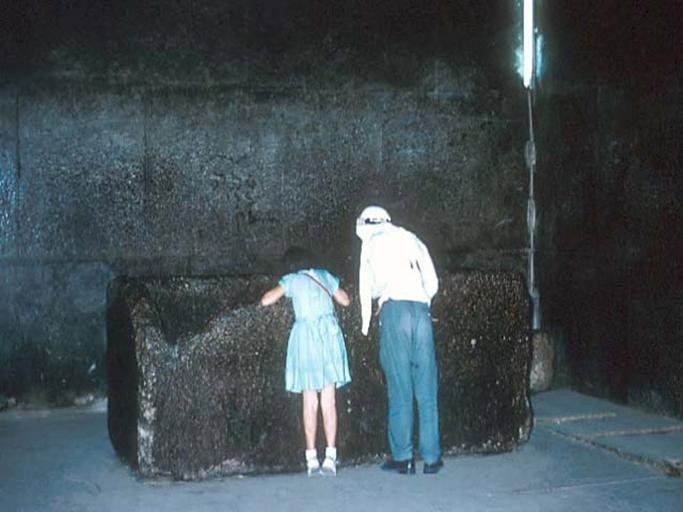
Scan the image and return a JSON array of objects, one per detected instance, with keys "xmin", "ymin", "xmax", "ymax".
[
  {"xmin": 353, "ymin": 200, "xmax": 445, "ymax": 478},
  {"xmin": 257, "ymin": 242, "xmax": 354, "ymax": 479}
]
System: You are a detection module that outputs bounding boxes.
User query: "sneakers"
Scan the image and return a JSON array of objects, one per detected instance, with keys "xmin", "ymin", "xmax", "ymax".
[
  {"xmin": 304, "ymin": 458, "xmax": 319, "ymax": 478},
  {"xmin": 318, "ymin": 457, "xmax": 337, "ymax": 477}
]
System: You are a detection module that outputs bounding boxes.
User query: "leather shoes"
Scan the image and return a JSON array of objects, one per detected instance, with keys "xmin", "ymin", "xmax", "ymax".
[
  {"xmin": 424, "ymin": 459, "xmax": 444, "ymax": 474},
  {"xmin": 380, "ymin": 457, "xmax": 416, "ymax": 474}
]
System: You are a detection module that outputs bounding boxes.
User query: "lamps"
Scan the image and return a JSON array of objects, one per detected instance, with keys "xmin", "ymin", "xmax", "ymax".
[{"xmin": 518, "ymin": 0, "xmax": 556, "ymax": 393}]
